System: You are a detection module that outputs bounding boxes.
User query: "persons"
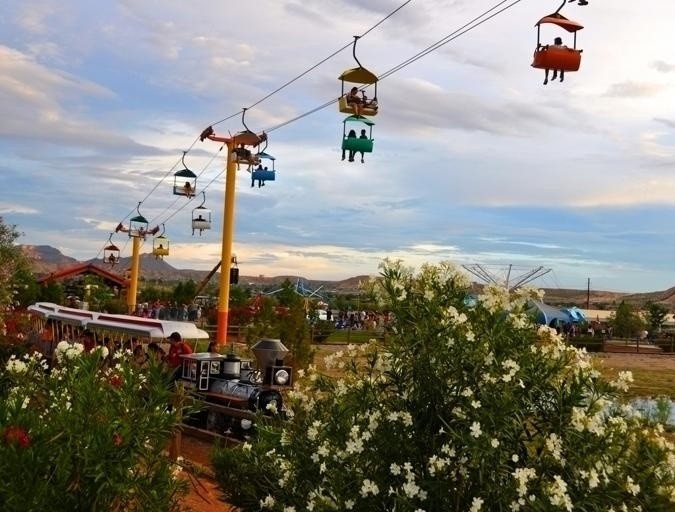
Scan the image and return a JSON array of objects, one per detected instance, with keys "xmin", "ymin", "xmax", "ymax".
[
  {"xmin": 155, "ymin": 244, "xmax": 163, "ymax": 261},
  {"xmin": 542, "ymin": 35, "xmax": 562, "ymax": 85},
  {"xmin": 109, "ymin": 253, "xmax": 115, "ymax": 267},
  {"xmin": 207, "ymin": 341, "xmax": 220, "ymax": 353},
  {"xmin": 560, "ymin": 44, "xmax": 568, "ymax": 83},
  {"xmin": 191, "ymin": 215, "xmax": 205, "ymax": 236},
  {"xmin": 575, "ymin": 325, "xmax": 579, "ymax": 333},
  {"xmin": 135, "ymin": 299, "xmax": 202, "ymax": 321},
  {"xmin": 260, "ymin": 166, "xmax": 268, "ymax": 186},
  {"xmin": 587, "ymin": 325, "xmax": 593, "ymax": 336},
  {"xmin": 348, "ymin": 87, "xmax": 364, "ymax": 115},
  {"xmin": 39, "ymin": 315, "xmax": 165, "ymax": 376},
  {"xmin": 341, "ymin": 129, "xmax": 355, "ymax": 161},
  {"xmin": 184, "ymin": 182, "xmax": 192, "ymax": 198},
  {"xmin": 607, "ymin": 326, "xmax": 612, "ymax": 335},
  {"xmin": 233, "ymin": 143, "xmax": 252, "ymax": 173},
  {"xmin": 40, "ymin": 319, "xmax": 45, "ymax": 320},
  {"xmin": 138, "ymin": 226, "xmax": 146, "ymax": 241},
  {"xmin": 352, "ymin": 129, "xmax": 368, "ymax": 162},
  {"xmin": 164, "ymin": 332, "xmax": 192, "ymax": 389},
  {"xmin": 328, "ymin": 305, "xmax": 391, "ymax": 328},
  {"xmin": 361, "ymin": 96, "xmax": 379, "ymax": 111},
  {"xmin": 250, "ymin": 164, "xmax": 263, "ymax": 187},
  {"xmin": 571, "ymin": 325, "xmax": 575, "ymax": 336}
]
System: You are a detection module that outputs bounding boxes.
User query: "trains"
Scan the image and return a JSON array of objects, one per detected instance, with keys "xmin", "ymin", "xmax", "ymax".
[{"xmin": 26, "ymin": 303, "xmax": 294, "ymax": 448}]
